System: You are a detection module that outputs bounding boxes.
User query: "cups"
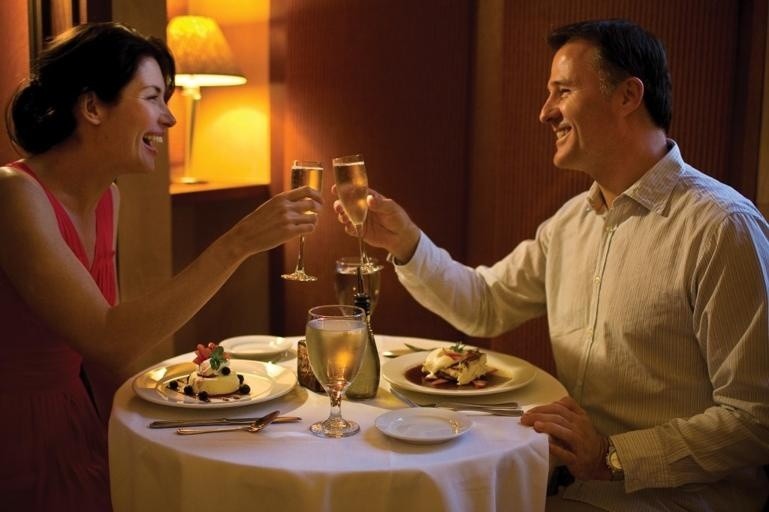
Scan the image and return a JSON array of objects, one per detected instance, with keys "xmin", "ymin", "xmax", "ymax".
[{"xmin": 297, "ymin": 336, "xmax": 325, "ymax": 393}]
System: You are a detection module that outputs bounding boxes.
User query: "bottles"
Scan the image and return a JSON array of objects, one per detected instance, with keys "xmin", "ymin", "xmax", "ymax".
[{"xmin": 340, "ymin": 266, "xmax": 380, "ymax": 400}]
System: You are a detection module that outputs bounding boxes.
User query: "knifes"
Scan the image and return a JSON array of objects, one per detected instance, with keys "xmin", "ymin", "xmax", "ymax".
[{"xmin": 147, "ymin": 412, "xmax": 304, "ymax": 430}]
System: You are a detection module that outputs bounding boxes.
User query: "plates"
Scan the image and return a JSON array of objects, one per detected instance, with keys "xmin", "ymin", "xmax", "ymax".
[
  {"xmin": 217, "ymin": 331, "xmax": 290, "ymax": 360},
  {"xmin": 373, "ymin": 407, "xmax": 479, "ymax": 446},
  {"xmin": 133, "ymin": 354, "xmax": 296, "ymax": 409},
  {"xmin": 384, "ymin": 347, "xmax": 536, "ymax": 398}
]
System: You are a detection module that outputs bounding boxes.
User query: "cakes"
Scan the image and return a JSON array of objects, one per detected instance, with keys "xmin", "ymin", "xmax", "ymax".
[
  {"xmin": 422, "ymin": 347, "xmax": 489, "ymax": 385},
  {"xmin": 188, "ymin": 342, "xmax": 242, "ymax": 397}
]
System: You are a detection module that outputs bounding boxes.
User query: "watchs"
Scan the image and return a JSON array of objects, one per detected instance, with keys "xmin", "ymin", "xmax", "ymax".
[{"xmin": 605, "ymin": 443, "xmax": 626, "ymax": 480}]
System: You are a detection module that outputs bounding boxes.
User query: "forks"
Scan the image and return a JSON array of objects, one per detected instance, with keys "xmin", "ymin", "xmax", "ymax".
[{"xmin": 390, "ymin": 389, "xmax": 527, "ymax": 418}]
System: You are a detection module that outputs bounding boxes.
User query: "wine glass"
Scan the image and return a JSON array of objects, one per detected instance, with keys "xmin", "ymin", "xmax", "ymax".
[
  {"xmin": 306, "ymin": 304, "xmax": 367, "ymax": 440},
  {"xmin": 331, "ymin": 258, "xmax": 385, "ymax": 324},
  {"xmin": 280, "ymin": 158, "xmax": 323, "ymax": 284},
  {"xmin": 331, "ymin": 154, "xmax": 372, "ymax": 264}
]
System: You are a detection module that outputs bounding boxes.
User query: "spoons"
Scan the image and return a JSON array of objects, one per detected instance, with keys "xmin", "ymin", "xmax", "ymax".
[{"xmin": 172, "ymin": 412, "xmax": 278, "ymax": 436}]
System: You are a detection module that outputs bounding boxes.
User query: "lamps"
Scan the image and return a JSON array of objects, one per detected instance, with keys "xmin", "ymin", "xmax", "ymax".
[{"xmin": 164, "ymin": 15, "xmax": 248, "ymax": 184}]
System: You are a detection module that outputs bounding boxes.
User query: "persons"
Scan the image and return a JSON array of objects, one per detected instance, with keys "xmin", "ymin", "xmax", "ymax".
[
  {"xmin": 327, "ymin": 19, "xmax": 769, "ymax": 511},
  {"xmin": 0, "ymin": 19, "xmax": 325, "ymax": 510}
]
